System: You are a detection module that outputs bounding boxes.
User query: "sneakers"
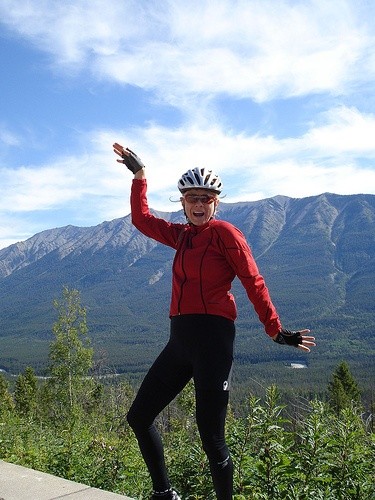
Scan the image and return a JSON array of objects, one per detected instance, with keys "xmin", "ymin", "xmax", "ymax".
[{"xmin": 142, "ymin": 492, "xmax": 181, "ymax": 500}]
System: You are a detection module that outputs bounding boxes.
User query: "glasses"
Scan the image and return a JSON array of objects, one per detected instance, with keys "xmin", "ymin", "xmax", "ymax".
[{"xmin": 182, "ymin": 194, "xmax": 217, "ymax": 203}]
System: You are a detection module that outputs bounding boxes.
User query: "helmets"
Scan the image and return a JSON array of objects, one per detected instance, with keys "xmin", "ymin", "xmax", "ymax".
[{"xmin": 177, "ymin": 168, "xmax": 223, "ymax": 195}]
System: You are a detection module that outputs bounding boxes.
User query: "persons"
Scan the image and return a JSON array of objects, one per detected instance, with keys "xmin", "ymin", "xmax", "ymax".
[{"xmin": 113, "ymin": 143, "xmax": 316, "ymax": 500}]
